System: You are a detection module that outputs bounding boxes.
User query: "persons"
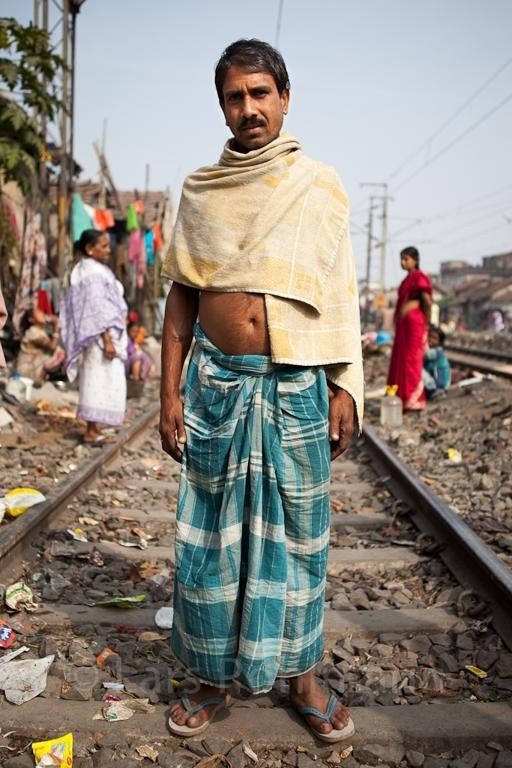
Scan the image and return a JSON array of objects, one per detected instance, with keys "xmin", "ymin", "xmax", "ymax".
[
  {"xmin": 361, "ymin": 298, "xmax": 511, "ymax": 403},
  {"xmin": 0, "ymin": 286, "xmax": 156, "ymax": 396},
  {"xmin": 57, "ymin": 229, "xmax": 131, "ymax": 445},
  {"xmin": 385, "ymin": 245, "xmax": 433, "ymax": 410},
  {"xmin": 155, "ymin": 36, "xmax": 372, "ymax": 746}
]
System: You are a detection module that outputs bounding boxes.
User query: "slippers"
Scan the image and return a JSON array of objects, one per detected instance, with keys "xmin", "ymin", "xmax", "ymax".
[
  {"xmin": 81, "ymin": 432, "xmax": 117, "ymax": 445},
  {"xmin": 296, "ymin": 690, "xmax": 356, "ymax": 743},
  {"xmin": 163, "ymin": 689, "xmax": 232, "ymax": 737}
]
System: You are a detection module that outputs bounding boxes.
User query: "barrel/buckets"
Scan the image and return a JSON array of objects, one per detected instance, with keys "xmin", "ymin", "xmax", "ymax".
[
  {"xmin": 9, "ymin": 377, "xmax": 34, "ymax": 401},
  {"xmin": 5, "ymin": 372, "xmax": 27, "ymax": 405},
  {"xmin": 380, "ymin": 384, "xmax": 403, "ymax": 427}
]
